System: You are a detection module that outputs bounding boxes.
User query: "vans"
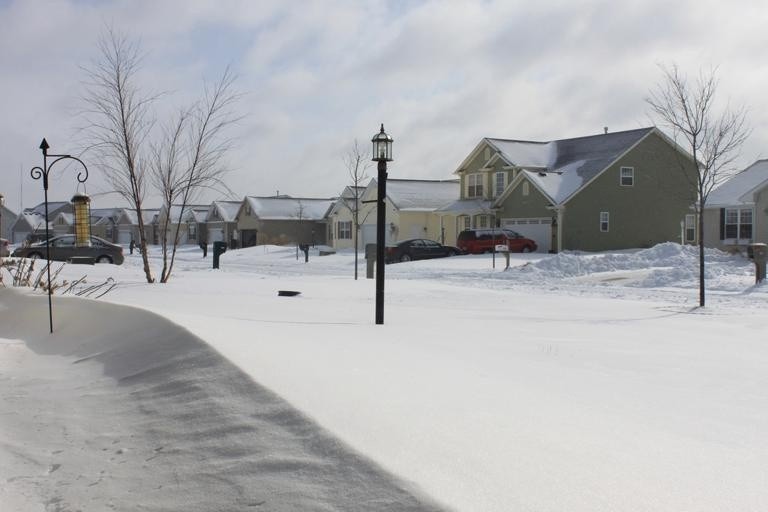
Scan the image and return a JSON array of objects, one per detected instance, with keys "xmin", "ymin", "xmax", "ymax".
[{"xmin": 456, "ymin": 227, "xmax": 538, "ymax": 255}]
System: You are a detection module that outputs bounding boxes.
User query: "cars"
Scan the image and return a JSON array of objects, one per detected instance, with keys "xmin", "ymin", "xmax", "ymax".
[
  {"xmin": 382, "ymin": 238, "xmax": 463, "ymax": 265},
  {"xmin": 11, "ymin": 231, "xmax": 126, "ymax": 265}
]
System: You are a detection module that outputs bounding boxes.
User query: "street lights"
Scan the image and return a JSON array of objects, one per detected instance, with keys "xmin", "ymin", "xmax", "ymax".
[
  {"xmin": 366, "ymin": 122, "xmax": 397, "ymax": 328},
  {"xmin": 25, "ymin": 137, "xmax": 99, "ymax": 335}
]
